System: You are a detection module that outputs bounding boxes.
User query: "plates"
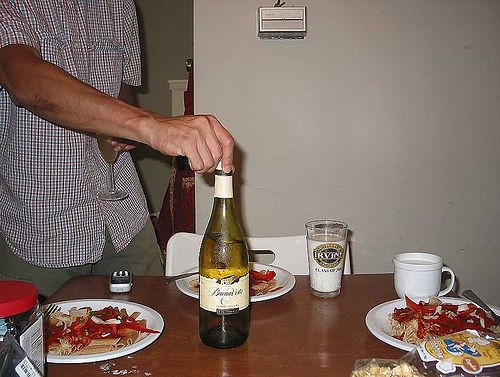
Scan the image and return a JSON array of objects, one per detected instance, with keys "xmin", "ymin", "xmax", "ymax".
[
  {"xmin": 44, "ymin": 299, "xmax": 165, "ymax": 364},
  {"xmin": 175, "ymin": 263, "xmax": 296, "ymax": 303},
  {"xmin": 365, "ymin": 296, "xmax": 500, "ymax": 352}
]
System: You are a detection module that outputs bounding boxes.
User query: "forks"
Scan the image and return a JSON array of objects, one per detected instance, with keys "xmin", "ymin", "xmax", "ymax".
[{"xmin": 43, "ymin": 304, "xmax": 61, "ymax": 318}]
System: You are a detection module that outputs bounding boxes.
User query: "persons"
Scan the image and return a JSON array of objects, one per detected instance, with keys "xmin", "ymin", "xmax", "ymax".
[{"xmin": 0, "ymin": 0, "xmax": 236, "ymax": 307}]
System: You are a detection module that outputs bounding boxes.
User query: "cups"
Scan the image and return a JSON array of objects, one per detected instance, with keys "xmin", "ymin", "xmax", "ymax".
[
  {"xmin": 306, "ymin": 220, "xmax": 348, "ymax": 298},
  {"xmin": 392, "ymin": 253, "xmax": 454, "ymax": 299}
]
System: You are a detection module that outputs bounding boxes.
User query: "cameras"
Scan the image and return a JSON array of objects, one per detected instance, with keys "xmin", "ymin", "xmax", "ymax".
[{"xmin": 109, "ymin": 269, "xmax": 133, "ymax": 292}]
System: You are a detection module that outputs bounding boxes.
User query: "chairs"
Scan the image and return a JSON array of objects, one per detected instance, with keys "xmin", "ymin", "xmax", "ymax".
[{"xmin": 165, "ymin": 232, "xmax": 351, "ymax": 277}]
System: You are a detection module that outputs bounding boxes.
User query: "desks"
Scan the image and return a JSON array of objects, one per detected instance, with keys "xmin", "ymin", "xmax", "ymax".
[{"xmin": 41, "ymin": 272, "xmax": 458, "ymax": 377}]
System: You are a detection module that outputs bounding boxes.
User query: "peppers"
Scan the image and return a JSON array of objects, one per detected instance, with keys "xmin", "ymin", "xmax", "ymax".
[
  {"xmin": 249, "ymin": 269, "xmax": 276, "ymax": 295},
  {"xmin": 394, "ymin": 293, "xmax": 500, "ymax": 338},
  {"xmin": 43, "ymin": 307, "xmax": 161, "ymax": 349}
]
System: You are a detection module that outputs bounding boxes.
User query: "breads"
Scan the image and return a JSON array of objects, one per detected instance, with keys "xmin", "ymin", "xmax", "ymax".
[{"xmin": 404, "ymin": 331, "xmax": 500, "ymax": 377}]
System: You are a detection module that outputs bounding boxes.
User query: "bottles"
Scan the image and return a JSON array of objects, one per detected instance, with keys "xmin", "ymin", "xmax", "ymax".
[
  {"xmin": 0, "ymin": 279, "xmax": 46, "ymax": 377},
  {"xmin": 199, "ymin": 160, "xmax": 249, "ymax": 348}
]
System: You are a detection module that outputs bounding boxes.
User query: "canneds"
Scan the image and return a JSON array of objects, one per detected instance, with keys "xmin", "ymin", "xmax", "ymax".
[{"xmin": 0, "ymin": 280, "xmax": 45, "ymax": 377}]
